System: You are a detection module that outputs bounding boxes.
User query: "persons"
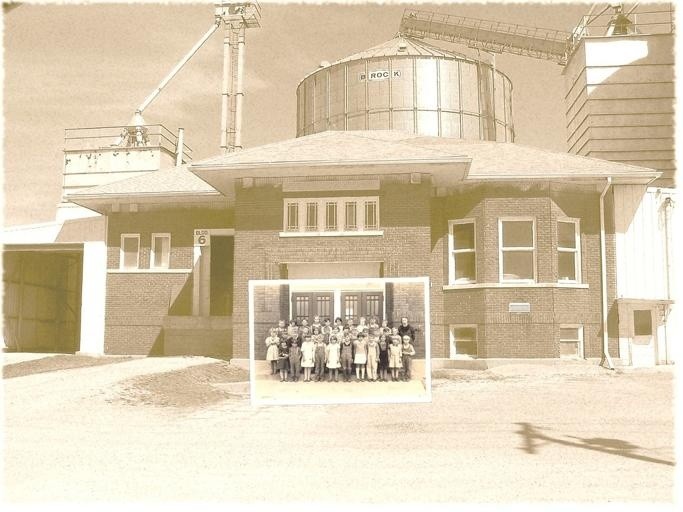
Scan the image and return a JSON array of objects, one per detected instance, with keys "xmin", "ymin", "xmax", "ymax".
[{"xmin": 263, "ymin": 313, "xmax": 416, "ymax": 383}]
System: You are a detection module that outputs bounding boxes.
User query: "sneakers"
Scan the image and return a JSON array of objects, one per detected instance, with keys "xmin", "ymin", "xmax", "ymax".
[{"xmin": 279, "ymin": 377, "xmax": 410, "ymax": 382}]
